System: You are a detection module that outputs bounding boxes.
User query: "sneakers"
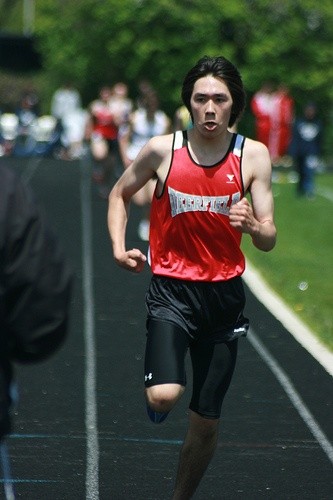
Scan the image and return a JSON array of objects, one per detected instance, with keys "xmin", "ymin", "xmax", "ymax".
[{"xmin": 146, "ymin": 405, "xmax": 170, "ymax": 424}]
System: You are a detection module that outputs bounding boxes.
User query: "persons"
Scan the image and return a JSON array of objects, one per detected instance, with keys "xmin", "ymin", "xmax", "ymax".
[
  {"xmin": 0, "ymin": 75, "xmax": 323, "ymax": 205},
  {"xmin": 107, "ymin": 55, "xmax": 278, "ymax": 500},
  {"xmin": 0, "ymin": 165, "xmax": 76, "ymax": 500}
]
